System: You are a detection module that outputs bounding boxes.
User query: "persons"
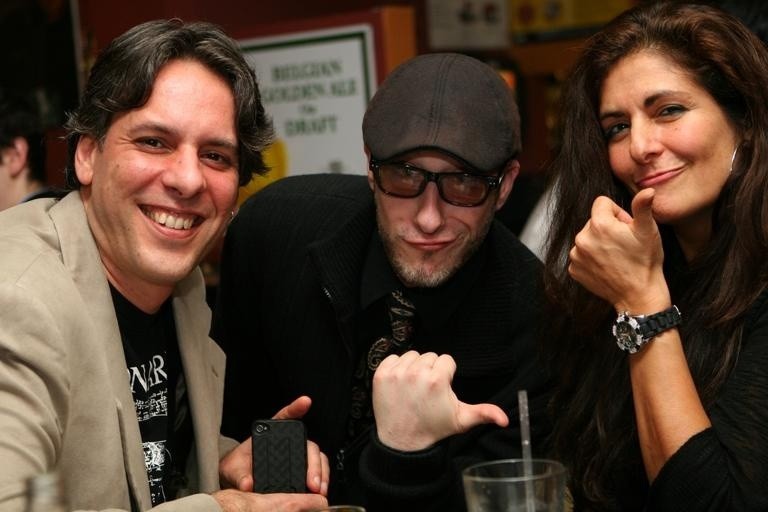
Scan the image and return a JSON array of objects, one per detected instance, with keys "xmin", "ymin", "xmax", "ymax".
[
  {"xmin": 0, "ymin": 19, "xmax": 332, "ymax": 512},
  {"xmin": 542, "ymin": 0, "xmax": 768, "ymax": 512},
  {"xmin": 0, "ymin": 83, "xmax": 72, "ymax": 211},
  {"xmin": 207, "ymin": 52, "xmax": 562, "ymax": 512}
]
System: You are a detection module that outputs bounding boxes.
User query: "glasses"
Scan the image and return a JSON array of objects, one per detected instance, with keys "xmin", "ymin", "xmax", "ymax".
[{"xmin": 370, "ymin": 154, "xmax": 502, "ymax": 208}]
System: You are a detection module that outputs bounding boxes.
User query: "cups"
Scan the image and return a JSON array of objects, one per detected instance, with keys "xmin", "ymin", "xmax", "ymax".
[{"xmin": 461, "ymin": 458, "xmax": 563, "ymax": 510}]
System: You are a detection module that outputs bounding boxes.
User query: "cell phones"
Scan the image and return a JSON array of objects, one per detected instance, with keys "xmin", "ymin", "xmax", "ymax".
[{"xmin": 251, "ymin": 420, "xmax": 308, "ymax": 494}]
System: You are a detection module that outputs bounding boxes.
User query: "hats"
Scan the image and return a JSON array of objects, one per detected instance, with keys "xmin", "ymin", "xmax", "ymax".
[{"xmin": 362, "ymin": 53, "xmax": 524, "ymax": 174}]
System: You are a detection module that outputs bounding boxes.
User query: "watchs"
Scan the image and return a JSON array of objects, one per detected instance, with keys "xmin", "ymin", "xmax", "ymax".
[{"xmin": 608, "ymin": 303, "xmax": 686, "ymax": 353}]
